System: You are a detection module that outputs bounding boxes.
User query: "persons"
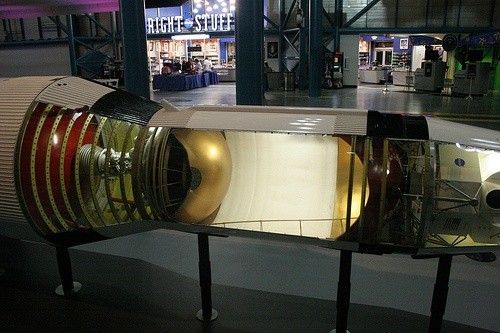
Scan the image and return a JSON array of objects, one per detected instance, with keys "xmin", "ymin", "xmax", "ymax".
[
  {"xmin": 204, "ymin": 57, "xmax": 211, "ymax": 71},
  {"xmin": 172, "ymin": 58, "xmax": 181, "ymax": 74},
  {"xmin": 183, "ymin": 57, "xmax": 193, "ymax": 72},
  {"xmin": 162, "ymin": 62, "xmax": 171, "ymax": 75},
  {"xmin": 194, "ymin": 58, "xmax": 201, "ymax": 74}
]
